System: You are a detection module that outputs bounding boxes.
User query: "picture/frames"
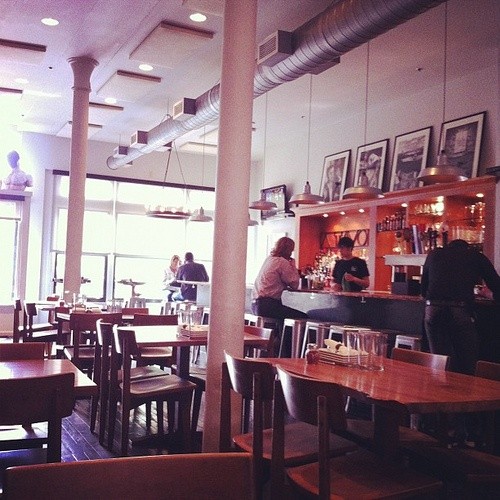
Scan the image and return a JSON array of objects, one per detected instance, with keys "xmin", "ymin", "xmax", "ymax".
[
  {"xmin": 260, "ymin": 184, "xmax": 288, "ymax": 221},
  {"xmin": 267, "ymin": 232, "xmax": 287, "ymax": 254},
  {"xmin": 436, "ymin": 110, "xmax": 487, "ymax": 180},
  {"xmin": 318, "ymin": 149, "xmax": 351, "ymax": 204},
  {"xmin": 351, "ymin": 138, "xmax": 390, "ymax": 192},
  {"xmin": 387, "ymin": 126, "xmax": 433, "ymax": 193}
]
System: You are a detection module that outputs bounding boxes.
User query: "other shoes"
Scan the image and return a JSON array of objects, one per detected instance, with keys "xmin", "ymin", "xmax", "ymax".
[{"xmin": 446, "ymin": 432, "xmax": 475, "ymax": 450}]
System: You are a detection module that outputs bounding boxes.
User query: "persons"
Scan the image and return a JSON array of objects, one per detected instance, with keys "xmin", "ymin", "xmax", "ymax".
[
  {"xmin": 251, "ymin": 237, "xmax": 310, "ymax": 352},
  {"xmin": 323, "ymin": 165, "xmax": 341, "ymax": 203},
  {"xmin": 420, "ymin": 239, "xmax": 500, "ymax": 375},
  {"xmin": 164, "ymin": 252, "xmax": 209, "ymax": 301},
  {"xmin": 330, "ymin": 236, "xmax": 370, "ymax": 292}
]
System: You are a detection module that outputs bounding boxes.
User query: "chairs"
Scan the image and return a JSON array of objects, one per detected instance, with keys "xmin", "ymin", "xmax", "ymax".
[{"xmin": 0, "ymin": 298, "xmax": 500, "ymax": 500}]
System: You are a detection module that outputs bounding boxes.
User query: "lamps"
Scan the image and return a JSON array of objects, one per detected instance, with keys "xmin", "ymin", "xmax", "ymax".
[
  {"xmin": 341, "ymin": 37, "xmax": 386, "ymax": 201},
  {"xmin": 412, "ymin": 1, "xmax": 470, "ymax": 187},
  {"xmin": 143, "ymin": 140, "xmax": 194, "ymax": 221},
  {"xmin": 250, "ymin": 92, "xmax": 278, "ymax": 210},
  {"xmin": 187, "ymin": 125, "xmax": 213, "ymax": 222},
  {"xmin": 247, "ymin": 213, "xmax": 259, "ymax": 227},
  {"xmin": 286, "ymin": 72, "xmax": 325, "ymax": 204}
]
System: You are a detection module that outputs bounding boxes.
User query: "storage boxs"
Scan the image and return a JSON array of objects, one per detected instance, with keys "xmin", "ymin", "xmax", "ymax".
[{"xmin": 391, "ymin": 281, "xmax": 420, "ymax": 296}]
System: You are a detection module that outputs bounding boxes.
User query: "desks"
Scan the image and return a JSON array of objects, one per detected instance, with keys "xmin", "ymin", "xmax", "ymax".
[
  {"xmin": 118, "ymin": 324, "xmax": 270, "ymax": 447},
  {"xmin": 245, "ymin": 354, "xmax": 500, "ymax": 500},
  {"xmin": 0, "ymin": 358, "xmax": 97, "ymax": 464},
  {"xmin": 57, "ymin": 312, "xmax": 134, "ymax": 344}
]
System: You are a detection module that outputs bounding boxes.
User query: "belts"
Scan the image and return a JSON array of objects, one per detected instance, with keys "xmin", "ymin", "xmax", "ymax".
[
  {"xmin": 425, "ymin": 299, "xmax": 470, "ymax": 307},
  {"xmin": 251, "ymin": 298, "xmax": 273, "ymax": 303}
]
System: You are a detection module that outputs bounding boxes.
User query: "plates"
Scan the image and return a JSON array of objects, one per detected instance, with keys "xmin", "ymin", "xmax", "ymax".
[
  {"xmin": 317, "ymin": 346, "xmax": 369, "ymax": 365},
  {"xmin": 178, "ymin": 323, "xmax": 209, "ymax": 338}
]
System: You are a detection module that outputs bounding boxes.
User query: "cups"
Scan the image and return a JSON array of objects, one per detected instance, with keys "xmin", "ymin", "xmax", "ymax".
[
  {"xmin": 345, "ymin": 331, "xmax": 388, "ymax": 370},
  {"xmin": 412, "ymin": 200, "xmax": 486, "ymax": 244},
  {"xmin": 177, "ymin": 304, "xmax": 206, "ymax": 327}
]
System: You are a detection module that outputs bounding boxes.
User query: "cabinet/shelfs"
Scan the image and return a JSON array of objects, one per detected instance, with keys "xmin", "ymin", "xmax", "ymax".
[{"xmin": 291, "ymin": 173, "xmax": 500, "ymax": 293}]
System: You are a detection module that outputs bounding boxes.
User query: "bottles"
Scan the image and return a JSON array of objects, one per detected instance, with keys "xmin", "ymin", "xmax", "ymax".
[
  {"xmin": 305, "ymin": 343, "xmax": 319, "ymax": 364},
  {"xmin": 377, "ymin": 210, "xmax": 405, "ymax": 232}
]
